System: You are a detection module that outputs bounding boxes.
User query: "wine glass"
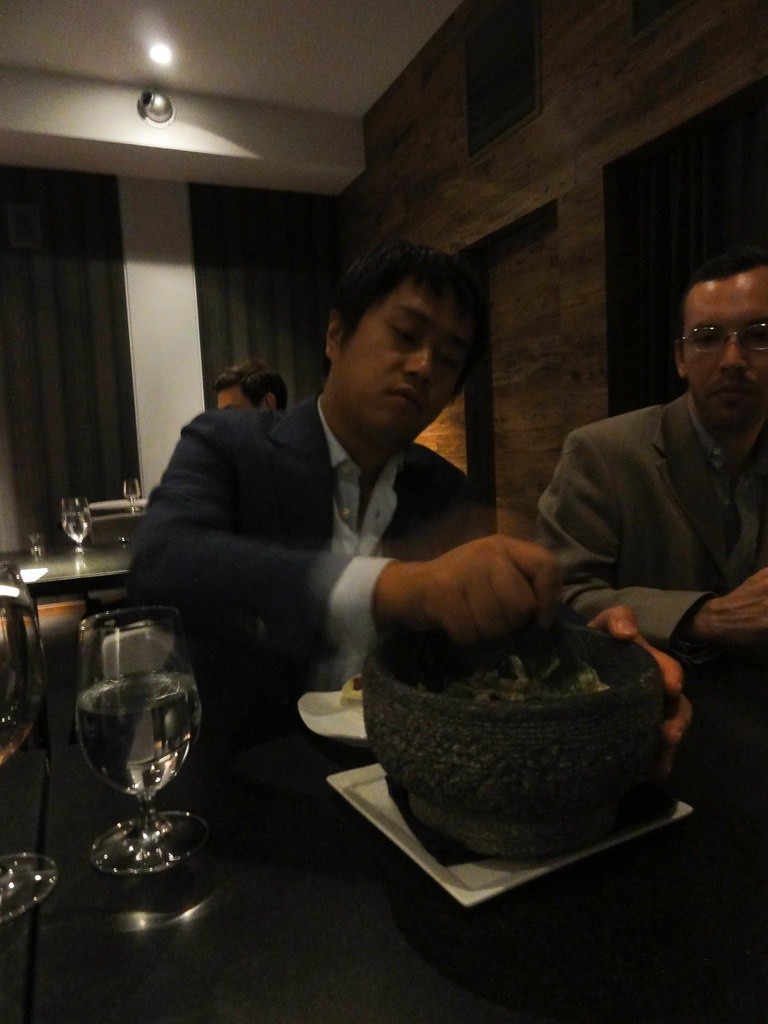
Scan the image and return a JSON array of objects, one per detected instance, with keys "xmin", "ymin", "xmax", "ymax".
[
  {"xmin": 0, "ymin": 560, "xmax": 58, "ymax": 924},
  {"xmin": 60, "ymin": 496, "xmax": 93, "ymax": 554},
  {"xmin": 123, "ymin": 478, "xmax": 142, "ymax": 516},
  {"xmin": 72, "ymin": 604, "xmax": 208, "ymax": 875}
]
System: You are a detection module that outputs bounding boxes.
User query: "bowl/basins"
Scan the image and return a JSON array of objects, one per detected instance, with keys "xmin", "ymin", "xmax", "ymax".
[{"xmin": 361, "ymin": 627, "xmax": 664, "ymax": 864}]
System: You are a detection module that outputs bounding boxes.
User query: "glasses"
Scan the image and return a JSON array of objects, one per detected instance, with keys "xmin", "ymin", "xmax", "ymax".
[{"xmin": 681, "ymin": 324, "xmax": 768, "ymax": 353}]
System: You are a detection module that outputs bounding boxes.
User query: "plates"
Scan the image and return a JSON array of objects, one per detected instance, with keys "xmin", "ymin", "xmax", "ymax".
[
  {"xmin": 325, "ymin": 762, "xmax": 695, "ymax": 908},
  {"xmin": 296, "ymin": 688, "xmax": 373, "ymax": 750}
]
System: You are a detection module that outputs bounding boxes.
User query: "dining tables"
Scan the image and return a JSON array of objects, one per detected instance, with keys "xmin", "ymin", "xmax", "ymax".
[{"xmin": 32, "ymin": 640, "xmax": 768, "ymax": 1024}]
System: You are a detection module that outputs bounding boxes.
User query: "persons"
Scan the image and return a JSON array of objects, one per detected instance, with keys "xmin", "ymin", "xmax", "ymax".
[
  {"xmin": 532, "ymin": 249, "xmax": 767, "ymax": 786},
  {"xmin": 212, "ymin": 358, "xmax": 288, "ymax": 413},
  {"xmin": 120, "ymin": 238, "xmax": 694, "ymax": 776}
]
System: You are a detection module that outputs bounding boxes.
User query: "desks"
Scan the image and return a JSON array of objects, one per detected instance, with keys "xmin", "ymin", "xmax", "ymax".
[
  {"xmin": 0, "ymin": 496, "xmax": 149, "ymax": 616},
  {"xmin": 0, "ymin": 749, "xmax": 47, "ymax": 1024}
]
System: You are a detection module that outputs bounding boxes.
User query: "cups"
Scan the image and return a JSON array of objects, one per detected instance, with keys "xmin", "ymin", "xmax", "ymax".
[{"xmin": 23, "ymin": 533, "xmax": 47, "ymax": 561}]
